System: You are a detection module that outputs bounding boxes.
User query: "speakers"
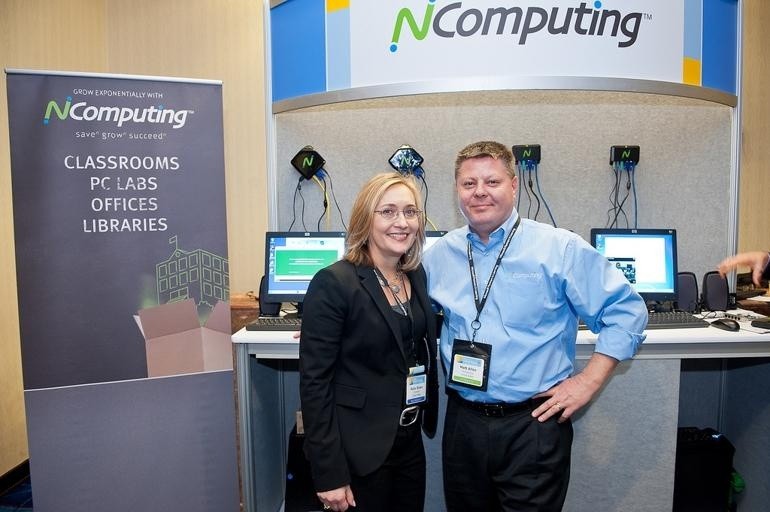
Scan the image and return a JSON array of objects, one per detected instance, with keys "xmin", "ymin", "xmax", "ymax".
[
  {"xmin": 674, "ymin": 272, "xmax": 698, "ymax": 311},
  {"xmin": 703, "ymin": 271, "xmax": 729, "ymax": 311}
]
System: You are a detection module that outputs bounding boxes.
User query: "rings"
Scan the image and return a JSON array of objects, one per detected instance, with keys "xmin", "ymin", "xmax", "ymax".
[
  {"xmin": 324, "ymin": 503, "xmax": 331, "ymax": 509},
  {"xmin": 556, "ymin": 403, "xmax": 563, "ymax": 411}
]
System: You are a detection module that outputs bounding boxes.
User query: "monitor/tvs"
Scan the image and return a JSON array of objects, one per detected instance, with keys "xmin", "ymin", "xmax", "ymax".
[
  {"xmin": 423, "ymin": 231, "xmax": 448, "ymax": 253},
  {"xmin": 264, "ymin": 232, "xmax": 348, "ymax": 318},
  {"xmin": 591, "ymin": 228, "xmax": 679, "ymax": 302}
]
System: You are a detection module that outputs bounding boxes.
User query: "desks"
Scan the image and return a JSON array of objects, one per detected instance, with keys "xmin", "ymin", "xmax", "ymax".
[{"xmin": 232, "ymin": 310, "xmax": 769, "ymax": 512}]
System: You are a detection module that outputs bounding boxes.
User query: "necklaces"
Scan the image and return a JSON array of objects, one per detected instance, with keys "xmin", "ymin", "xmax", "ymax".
[{"xmin": 370, "ymin": 264, "xmax": 405, "ymax": 294}]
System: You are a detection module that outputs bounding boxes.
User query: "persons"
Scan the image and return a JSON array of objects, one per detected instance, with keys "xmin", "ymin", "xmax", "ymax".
[
  {"xmin": 414, "ymin": 139, "xmax": 651, "ymax": 512},
  {"xmin": 716, "ymin": 248, "xmax": 769, "ymax": 289},
  {"xmin": 282, "ymin": 172, "xmax": 447, "ymax": 510}
]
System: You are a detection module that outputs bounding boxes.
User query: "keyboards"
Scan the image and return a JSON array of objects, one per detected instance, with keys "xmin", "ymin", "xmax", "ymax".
[
  {"xmin": 246, "ymin": 319, "xmax": 302, "ymax": 331},
  {"xmin": 645, "ymin": 311, "xmax": 710, "ymax": 328}
]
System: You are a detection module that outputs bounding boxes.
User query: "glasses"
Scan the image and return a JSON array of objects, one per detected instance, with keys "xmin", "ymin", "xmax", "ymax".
[{"xmin": 373, "ymin": 207, "xmax": 423, "ymax": 220}]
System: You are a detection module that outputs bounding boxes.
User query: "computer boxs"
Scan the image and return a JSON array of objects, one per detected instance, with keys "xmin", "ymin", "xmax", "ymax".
[
  {"xmin": 674, "ymin": 427, "xmax": 735, "ymax": 512},
  {"xmin": 284, "ymin": 421, "xmax": 322, "ymax": 512}
]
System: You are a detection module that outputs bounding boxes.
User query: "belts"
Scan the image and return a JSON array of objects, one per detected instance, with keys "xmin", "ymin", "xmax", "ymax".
[
  {"xmin": 450, "ymin": 391, "xmax": 552, "ymax": 418},
  {"xmin": 399, "ymin": 405, "xmax": 420, "ymax": 427}
]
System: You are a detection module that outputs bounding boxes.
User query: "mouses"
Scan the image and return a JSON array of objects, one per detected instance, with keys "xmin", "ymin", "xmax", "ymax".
[{"xmin": 712, "ymin": 318, "xmax": 740, "ymax": 332}]
